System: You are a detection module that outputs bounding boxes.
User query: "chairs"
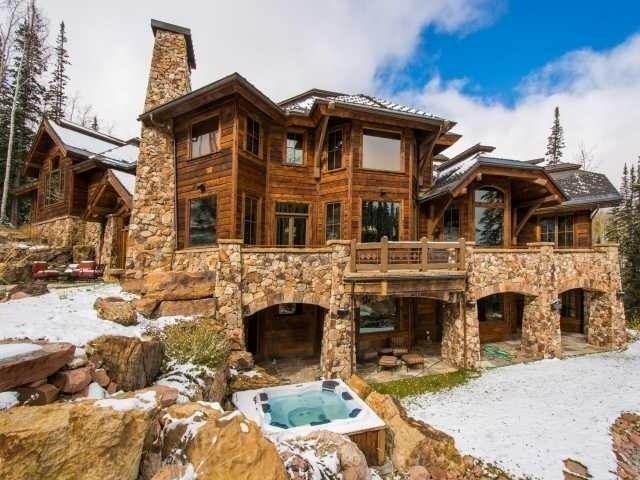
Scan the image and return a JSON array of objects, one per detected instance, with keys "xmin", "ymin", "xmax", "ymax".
[
  {"xmin": 358, "ymin": 340, "xmax": 379, "ymax": 360},
  {"xmin": 392, "ymin": 336, "xmax": 409, "ymax": 356}
]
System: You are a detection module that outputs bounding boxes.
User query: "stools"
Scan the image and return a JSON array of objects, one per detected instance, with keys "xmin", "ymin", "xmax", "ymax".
[
  {"xmin": 375, "ymin": 345, "xmax": 393, "ymax": 359},
  {"xmin": 377, "ymin": 355, "xmax": 400, "ymax": 373},
  {"xmin": 401, "ymin": 353, "xmax": 426, "ymax": 372}
]
